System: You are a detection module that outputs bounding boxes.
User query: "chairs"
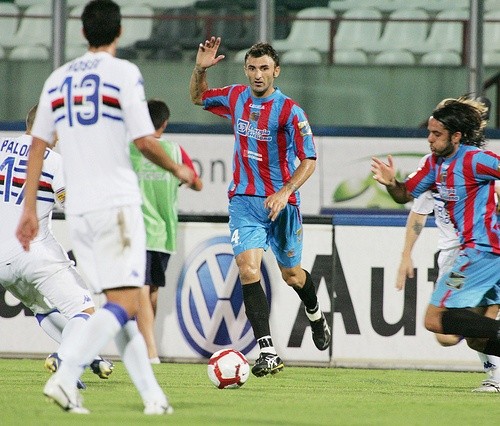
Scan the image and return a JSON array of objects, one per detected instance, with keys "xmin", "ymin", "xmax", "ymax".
[{"xmin": 0, "ymin": 0, "xmax": 500, "ymax": 67}]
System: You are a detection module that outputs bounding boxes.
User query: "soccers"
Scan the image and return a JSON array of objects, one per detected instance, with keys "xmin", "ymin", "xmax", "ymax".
[{"xmin": 208, "ymin": 348, "xmax": 249, "ymax": 389}]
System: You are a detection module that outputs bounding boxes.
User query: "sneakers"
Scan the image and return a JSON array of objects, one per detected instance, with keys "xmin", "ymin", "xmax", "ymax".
[
  {"xmin": 42, "ymin": 375, "xmax": 89, "ymax": 414},
  {"xmin": 89, "ymin": 356, "xmax": 114, "ymax": 380},
  {"xmin": 144, "ymin": 397, "xmax": 173, "ymax": 414},
  {"xmin": 251, "ymin": 352, "xmax": 284, "ymax": 377},
  {"xmin": 44, "ymin": 352, "xmax": 86, "ymax": 388},
  {"xmin": 472, "ymin": 380, "xmax": 500, "ymax": 392},
  {"xmin": 310, "ymin": 309, "xmax": 332, "ymax": 351}
]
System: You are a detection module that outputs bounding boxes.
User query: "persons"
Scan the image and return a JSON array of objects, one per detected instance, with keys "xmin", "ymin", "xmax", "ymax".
[
  {"xmin": 16, "ymin": 0, "xmax": 195, "ymax": 416},
  {"xmin": 128, "ymin": 99, "xmax": 203, "ymax": 363},
  {"xmin": 370, "ymin": 97, "xmax": 500, "ymax": 358},
  {"xmin": 0, "ymin": 105, "xmax": 114, "ymax": 388},
  {"xmin": 189, "ymin": 36, "xmax": 330, "ymax": 377},
  {"xmin": 394, "ymin": 179, "xmax": 500, "ymax": 394}
]
